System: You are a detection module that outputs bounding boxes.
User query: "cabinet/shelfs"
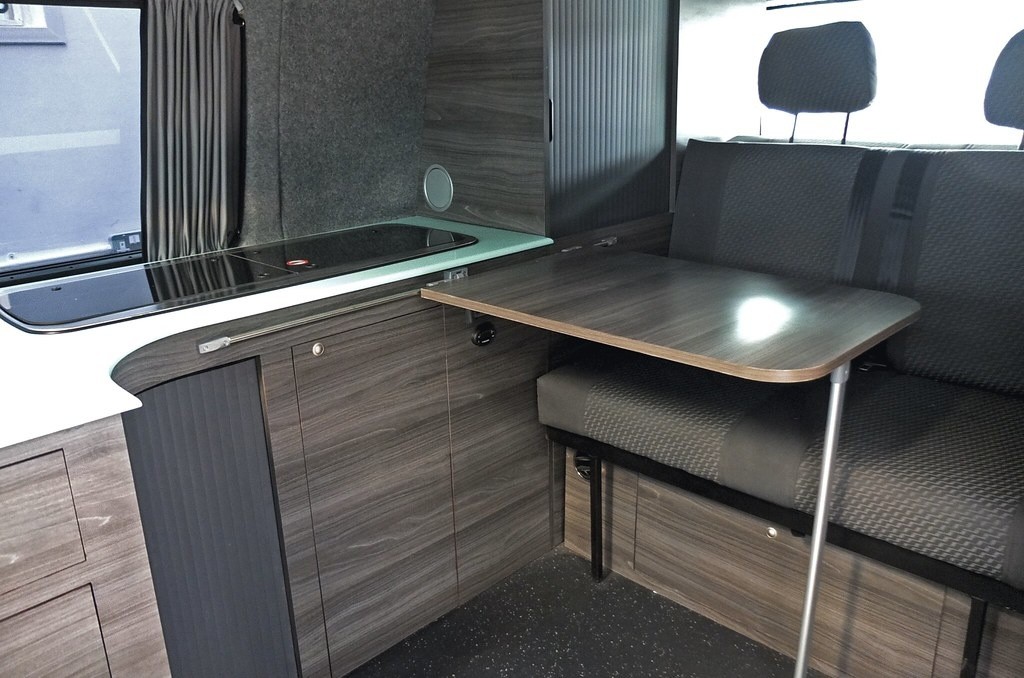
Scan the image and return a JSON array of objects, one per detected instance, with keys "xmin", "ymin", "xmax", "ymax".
[
  {"xmin": 0, "ymin": 449, "xmax": 113, "ymax": 678},
  {"xmin": 632, "ymin": 470, "xmax": 947, "ymax": 678},
  {"xmin": 292, "ymin": 305, "xmax": 553, "ymax": 678}
]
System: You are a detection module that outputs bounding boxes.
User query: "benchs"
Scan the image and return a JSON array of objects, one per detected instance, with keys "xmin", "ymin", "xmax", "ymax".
[{"xmin": 537, "ymin": 20, "xmax": 1024, "ymax": 678}]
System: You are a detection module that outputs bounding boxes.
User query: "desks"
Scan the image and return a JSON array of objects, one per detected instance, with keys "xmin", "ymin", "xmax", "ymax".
[{"xmin": 421, "ymin": 250, "xmax": 922, "ymax": 678}]
